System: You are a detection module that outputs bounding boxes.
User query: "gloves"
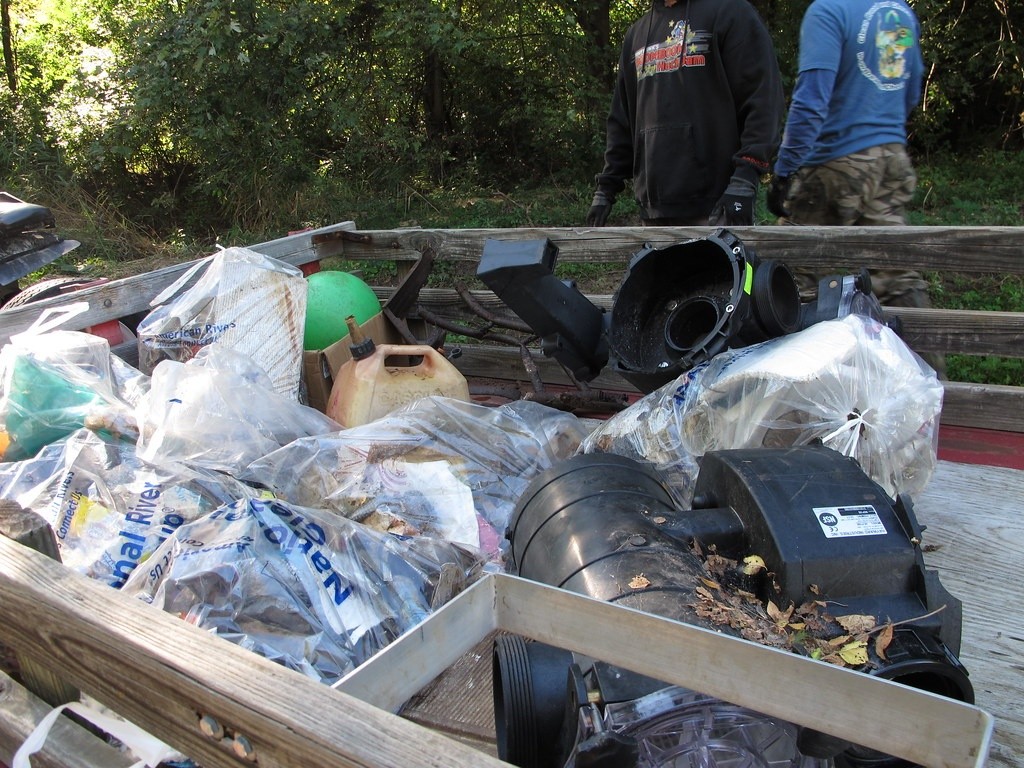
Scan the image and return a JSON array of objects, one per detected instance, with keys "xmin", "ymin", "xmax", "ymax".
[
  {"xmin": 766, "ymin": 174, "xmax": 794, "ymax": 218},
  {"xmin": 708, "ymin": 177, "xmax": 758, "ymax": 226},
  {"xmin": 584, "ymin": 190, "xmax": 618, "ymax": 227}
]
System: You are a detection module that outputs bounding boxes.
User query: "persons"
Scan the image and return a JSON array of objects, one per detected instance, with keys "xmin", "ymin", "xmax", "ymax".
[
  {"xmin": 584, "ymin": 1, "xmax": 784, "ymax": 226},
  {"xmin": 765, "ymin": 0, "xmax": 949, "ymax": 382}
]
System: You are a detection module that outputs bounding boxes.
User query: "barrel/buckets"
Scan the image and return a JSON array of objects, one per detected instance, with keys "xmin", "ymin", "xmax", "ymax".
[{"xmin": 326, "ymin": 315, "xmax": 471, "ymax": 431}]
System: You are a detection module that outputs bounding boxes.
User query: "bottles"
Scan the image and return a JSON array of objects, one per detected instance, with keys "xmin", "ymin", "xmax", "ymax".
[{"xmin": 777, "ymin": 174, "xmax": 802, "ymax": 225}]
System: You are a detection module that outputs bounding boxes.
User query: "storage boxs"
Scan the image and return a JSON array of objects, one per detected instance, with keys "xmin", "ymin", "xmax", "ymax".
[{"xmin": 303, "ymin": 299, "xmax": 410, "ymax": 415}]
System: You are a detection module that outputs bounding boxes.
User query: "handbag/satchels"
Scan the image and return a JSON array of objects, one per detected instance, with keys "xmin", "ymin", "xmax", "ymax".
[{"xmin": 0, "ymin": 242, "xmax": 944, "ymax": 685}]
